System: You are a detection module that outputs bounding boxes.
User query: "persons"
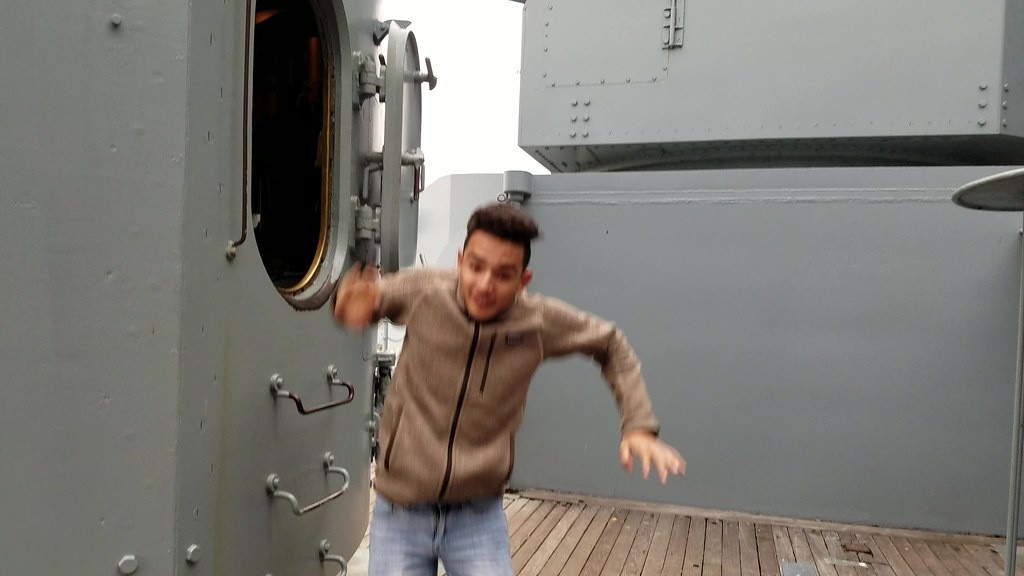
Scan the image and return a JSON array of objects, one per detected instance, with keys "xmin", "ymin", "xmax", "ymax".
[{"xmin": 334, "ymin": 203, "xmax": 686, "ymax": 576}]
